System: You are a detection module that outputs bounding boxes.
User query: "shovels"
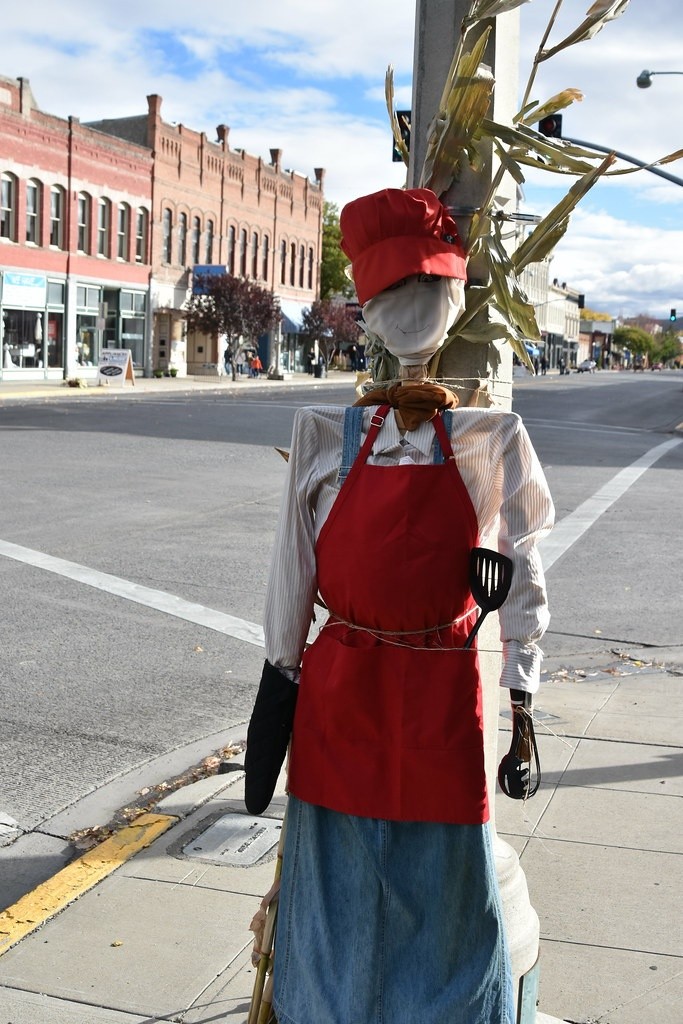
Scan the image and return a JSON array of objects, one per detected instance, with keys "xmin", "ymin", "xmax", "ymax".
[{"xmin": 464, "ymin": 546, "xmax": 512, "ymax": 649}]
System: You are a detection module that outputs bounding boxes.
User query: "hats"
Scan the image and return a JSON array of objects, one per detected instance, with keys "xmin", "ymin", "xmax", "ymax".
[{"xmin": 339, "ymin": 188, "xmax": 468, "ymax": 305}]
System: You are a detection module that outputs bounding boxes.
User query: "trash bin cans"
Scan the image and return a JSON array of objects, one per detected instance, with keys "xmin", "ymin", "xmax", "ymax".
[{"xmin": 314, "ymin": 364, "xmax": 326, "ymax": 378}]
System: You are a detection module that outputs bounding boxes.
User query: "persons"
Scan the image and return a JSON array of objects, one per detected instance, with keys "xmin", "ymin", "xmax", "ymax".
[
  {"xmin": 224, "ymin": 343, "xmax": 264, "ymax": 379},
  {"xmin": 307, "ymin": 346, "xmax": 316, "ymax": 376},
  {"xmin": 349, "ymin": 346, "xmax": 359, "ymax": 373},
  {"xmin": 532, "ymin": 356, "xmax": 572, "ymax": 376}
]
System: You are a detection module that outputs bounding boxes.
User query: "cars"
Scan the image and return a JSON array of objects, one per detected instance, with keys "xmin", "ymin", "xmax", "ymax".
[
  {"xmin": 652, "ymin": 362, "xmax": 663, "ymax": 372},
  {"xmin": 578, "ymin": 360, "xmax": 596, "ymax": 374}
]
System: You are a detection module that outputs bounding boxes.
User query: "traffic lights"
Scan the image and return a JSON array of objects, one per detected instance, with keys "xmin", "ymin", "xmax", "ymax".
[
  {"xmin": 538, "ymin": 113, "xmax": 563, "ymax": 166},
  {"xmin": 391, "ymin": 111, "xmax": 412, "ymax": 163},
  {"xmin": 670, "ymin": 309, "xmax": 676, "ymax": 322}
]
